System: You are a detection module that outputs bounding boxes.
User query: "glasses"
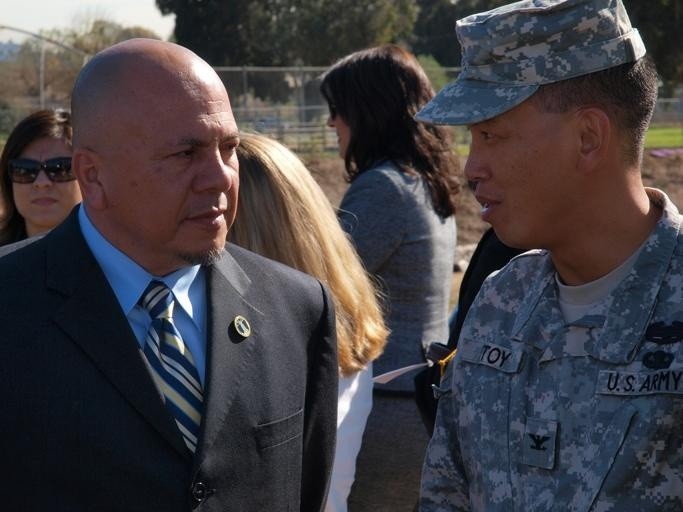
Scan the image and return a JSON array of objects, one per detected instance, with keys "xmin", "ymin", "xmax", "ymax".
[{"xmin": 8, "ymin": 158, "xmax": 76, "ymax": 184}]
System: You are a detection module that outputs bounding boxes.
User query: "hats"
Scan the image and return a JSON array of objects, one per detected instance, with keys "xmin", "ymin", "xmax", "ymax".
[{"xmin": 413, "ymin": 0, "xmax": 647, "ymax": 125}]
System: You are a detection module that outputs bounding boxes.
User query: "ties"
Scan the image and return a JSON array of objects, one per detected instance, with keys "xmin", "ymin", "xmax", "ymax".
[{"xmin": 138, "ymin": 281, "xmax": 204, "ymax": 454}]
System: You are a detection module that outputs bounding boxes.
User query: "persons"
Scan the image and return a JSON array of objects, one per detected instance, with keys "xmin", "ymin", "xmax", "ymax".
[
  {"xmin": 320, "ymin": 45, "xmax": 465, "ymax": 512},
  {"xmin": 419, "ymin": 0, "xmax": 683, "ymax": 512},
  {"xmin": 0, "ymin": 109, "xmax": 83, "ymax": 246},
  {"xmin": 415, "ymin": 182, "xmax": 528, "ymax": 442},
  {"xmin": 0, "ymin": 36, "xmax": 339, "ymax": 512},
  {"xmin": 226, "ymin": 130, "xmax": 391, "ymax": 512}
]
